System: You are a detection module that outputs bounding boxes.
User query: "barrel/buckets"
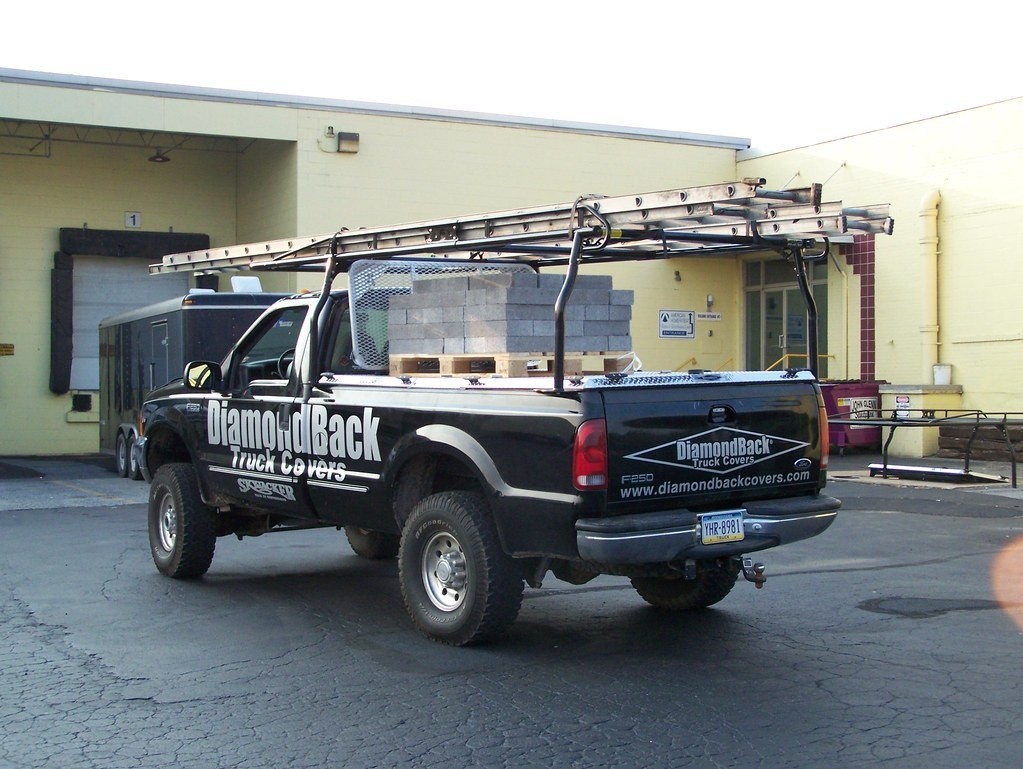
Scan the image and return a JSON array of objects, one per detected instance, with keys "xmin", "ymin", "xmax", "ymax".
[{"xmin": 933, "ymin": 363, "xmax": 950, "ymax": 385}]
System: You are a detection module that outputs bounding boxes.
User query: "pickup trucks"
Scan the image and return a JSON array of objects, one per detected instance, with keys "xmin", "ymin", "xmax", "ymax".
[{"xmin": 132, "ymin": 176, "xmax": 896, "ymax": 646}]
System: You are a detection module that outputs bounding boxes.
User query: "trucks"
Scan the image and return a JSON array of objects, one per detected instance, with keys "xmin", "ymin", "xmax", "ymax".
[{"xmin": 97, "ymin": 278, "xmax": 368, "ymax": 484}]
